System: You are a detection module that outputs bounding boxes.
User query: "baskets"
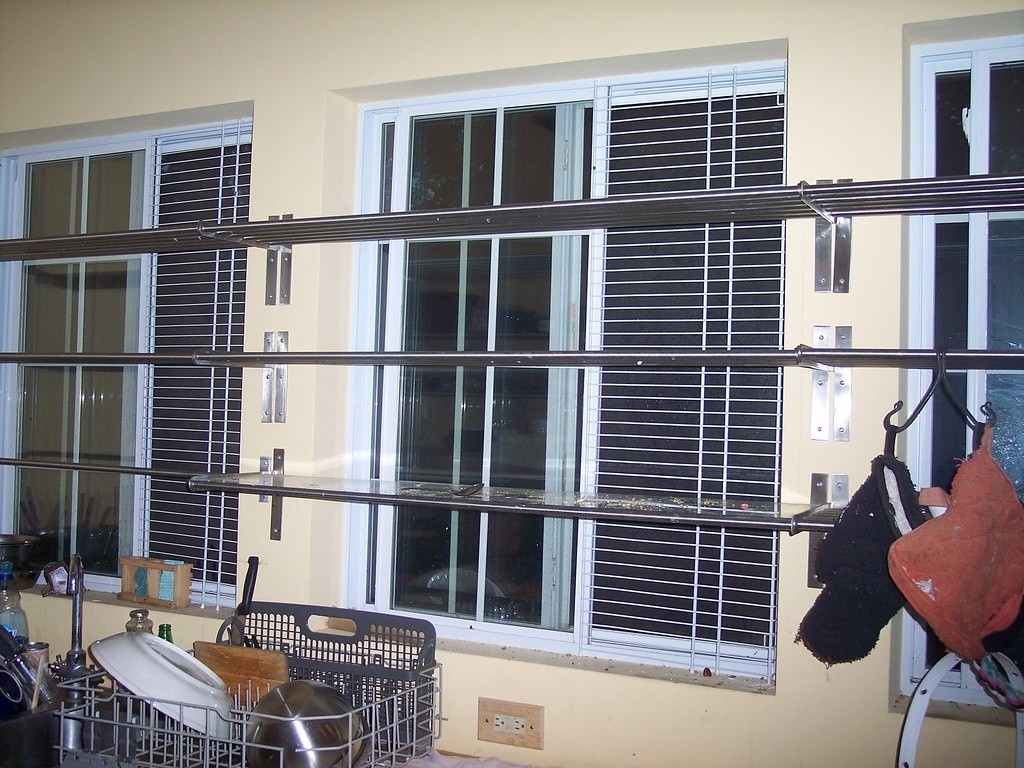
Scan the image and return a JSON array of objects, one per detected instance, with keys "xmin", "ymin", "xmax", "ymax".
[{"xmin": 54, "ymin": 640, "xmax": 448, "ymax": 768}]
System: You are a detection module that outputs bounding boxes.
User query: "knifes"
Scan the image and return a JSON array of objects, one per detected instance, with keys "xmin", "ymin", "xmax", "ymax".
[{"xmin": 225, "ymin": 557, "xmax": 258, "ymax": 645}]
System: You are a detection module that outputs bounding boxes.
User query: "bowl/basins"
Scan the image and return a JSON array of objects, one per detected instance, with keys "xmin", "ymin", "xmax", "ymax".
[
  {"xmin": 242, "ymin": 680, "xmax": 364, "ymax": 768},
  {"xmin": 0, "ymin": 541, "xmax": 49, "ymax": 591}
]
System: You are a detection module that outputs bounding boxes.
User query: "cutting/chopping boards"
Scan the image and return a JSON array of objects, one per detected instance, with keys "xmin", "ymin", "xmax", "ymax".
[{"xmin": 194, "ymin": 642, "xmax": 289, "ymax": 706}]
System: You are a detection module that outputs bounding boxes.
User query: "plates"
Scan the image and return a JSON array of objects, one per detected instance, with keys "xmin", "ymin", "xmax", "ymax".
[
  {"xmin": 92, "ymin": 632, "xmax": 234, "ymax": 739},
  {"xmin": 429, "ymin": 570, "xmax": 505, "ymax": 618}
]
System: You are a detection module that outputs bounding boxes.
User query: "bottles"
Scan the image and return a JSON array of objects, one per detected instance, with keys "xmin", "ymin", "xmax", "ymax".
[
  {"xmin": 158, "ymin": 624, "xmax": 173, "ymax": 643},
  {"xmin": 126, "ymin": 609, "xmax": 153, "ymax": 634},
  {"xmin": 0, "ymin": 561, "xmax": 29, "ymax": 644}
]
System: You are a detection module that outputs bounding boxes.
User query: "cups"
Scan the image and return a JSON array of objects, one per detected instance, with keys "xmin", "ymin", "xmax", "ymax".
[{"xmin": 6, "ymin": 652, "xmax": 56, "ymax": 701}]
